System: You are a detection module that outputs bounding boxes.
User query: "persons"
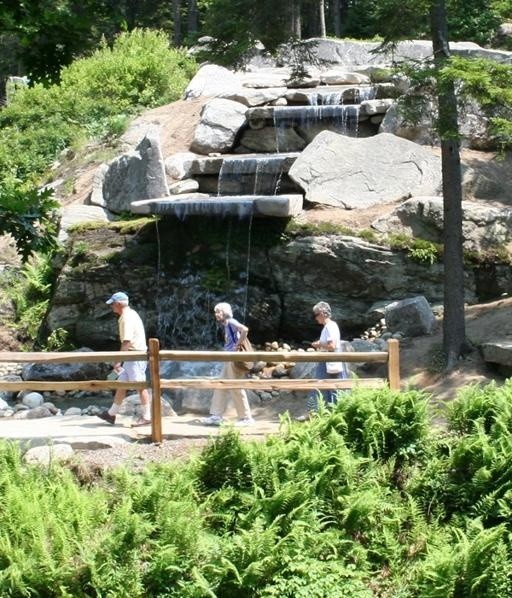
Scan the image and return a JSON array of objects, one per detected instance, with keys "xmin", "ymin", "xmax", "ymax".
[
  {"xmin": 294, "ymin": 300, "xmax": 351, "ymax": 422},
  {"xmin": 201, "ymin": 301, "xmax": 257, "ymax": 428},
  {"xmin": 91, "ymin": 291, "xmax": 152, "ymax": 428}
]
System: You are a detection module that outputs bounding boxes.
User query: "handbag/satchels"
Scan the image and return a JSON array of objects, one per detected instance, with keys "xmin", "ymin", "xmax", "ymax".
[
  {"xmin": 231, "ymin": 335, "xmax": 255, "ymax": 373},
  {"xmin": 325, "ymin": 361, "xmax": 343, "ymax": 374}
]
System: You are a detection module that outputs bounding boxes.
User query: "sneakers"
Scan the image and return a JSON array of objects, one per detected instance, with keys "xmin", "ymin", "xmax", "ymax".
[
  {"xmin": 95, "ymin": 409, "xmax": 116, "ymax": 424},
  {"xmin": 235, "ymin": 418, "xmax": 257, "ymax": 428},
  {"xmin": 198, "ymin": 415, "xmax": 223, "ymax": 426},
  {"xmin": 131, "ymin": 415, "xmax": 153, "ymax": 426},
  {"xmin": 296, "ymin": 415, "xmax": 307, "ymax": 422}
]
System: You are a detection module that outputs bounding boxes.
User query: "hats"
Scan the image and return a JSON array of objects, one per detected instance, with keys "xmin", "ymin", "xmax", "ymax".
[{"xmin": 105, "ymin": 291, "xmax": 130, "ymax": 304}]
